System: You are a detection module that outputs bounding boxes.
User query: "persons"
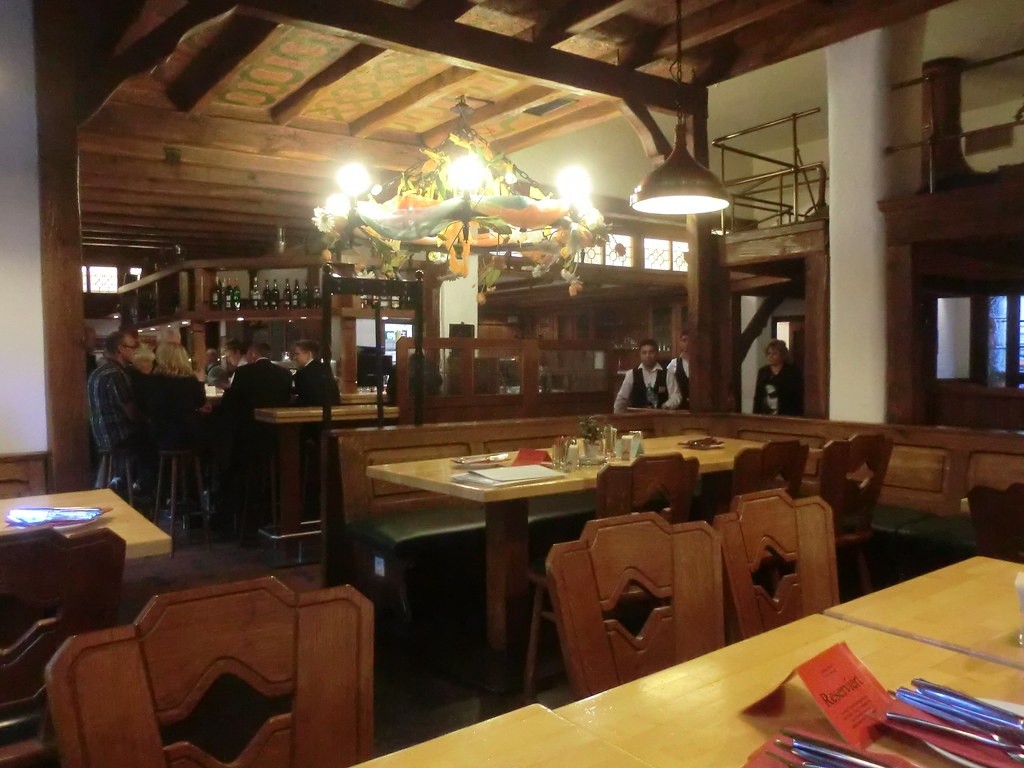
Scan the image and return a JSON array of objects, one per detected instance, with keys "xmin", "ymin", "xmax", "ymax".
[
  {"xmin": 82, "ymin": 321, "xmax": 341, "ymax": 525},
  {"xmin": 614, "ymin": 329, "xmax": 690, "ymax": 414},
  {"xmin": 752, "ymin": 341, "xmax": 805, "ymax": 417}
]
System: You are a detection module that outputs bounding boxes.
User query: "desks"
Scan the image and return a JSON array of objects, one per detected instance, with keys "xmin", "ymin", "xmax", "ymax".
[
  {"xmin": 554, "ymin": 611, "xmax": 1023, "ymax": 767},
  {"xmin": 366, "ymin": 434, "xmax": 767, "ymax": 694},
  {"xmin": 349, "ymin": 702, "xmax": 652, "ymax": 767},
  {"xmin": 0, "ymin": 487, "xmax": 175, "ymax": 558},
  {"xmin": 822, "ymin": 554, "xmax": 1024, "ymax": 670}
]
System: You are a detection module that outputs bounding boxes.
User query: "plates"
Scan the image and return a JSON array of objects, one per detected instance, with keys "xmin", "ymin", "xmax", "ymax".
[
  {"xmin": 6, "ymin": 507, "xmax": 99, "ymax": 532},
  {"xmin": 449, "ymin": 455, "xmax": 512, "ymax": 468}
]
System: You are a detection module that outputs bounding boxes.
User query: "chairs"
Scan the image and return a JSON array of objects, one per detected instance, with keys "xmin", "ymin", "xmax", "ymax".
[
  {"xmin": 0, "ymin": 526, "xmax": 125, "ymax": 768},
  {"xmin": 596, "ymin": 452, "xmax": 700, "ymax": 523},
  {"xmin": 546, "ymin": 511, "xmax": 725, "ymax": 699},
  {"xmin": 968, "ymin": 482, "xmax": 1024, "ymax": 564},
  {"xmin": 46, "ymin": 573, "xmax": 374, "ymax": 767},
  {"xmin": 816, "ymin": 432, "xmax": 894, "ymax": 593},
  {"xmin": 733, "ymin": 439, "xmax": 809, "ymax": 498},
  {"xmin": 715, "ymin": 488, "xmax": 840, "ymax": 642}
]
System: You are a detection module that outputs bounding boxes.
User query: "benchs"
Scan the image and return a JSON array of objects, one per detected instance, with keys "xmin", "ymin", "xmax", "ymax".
[{"xmin": 331, "ymin": 412, "xmax": 1023, "ymax": 638}]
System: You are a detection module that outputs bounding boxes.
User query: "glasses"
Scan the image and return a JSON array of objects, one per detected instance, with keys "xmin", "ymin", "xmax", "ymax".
[{"xmin": 123, "ymin": 345, "xmax": 137, "ymax": 351}]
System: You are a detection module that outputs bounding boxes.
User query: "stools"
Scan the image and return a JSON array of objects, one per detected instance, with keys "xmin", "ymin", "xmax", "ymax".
[
  {"xmin": 154, "ymin": 448, "xmax": 211, "ymax": 557},
  {"xmin": 92, "ymin": 445, "xmax": 136, "ymax": 507}
]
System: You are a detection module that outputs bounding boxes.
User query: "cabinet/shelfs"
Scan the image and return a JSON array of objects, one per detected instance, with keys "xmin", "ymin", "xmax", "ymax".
[{"xmin": 117, "ymin": 256, "xmax": 357, "ymax": 394}]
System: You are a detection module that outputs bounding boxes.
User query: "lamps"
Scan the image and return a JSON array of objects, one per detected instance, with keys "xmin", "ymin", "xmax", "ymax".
[
  {"xmin": 313, "ymin": 92, "xmax": 626, "ymax": 304},
  {"xmin": 629, "ymin": 0, "xmax": 730, "ymax": 215}
]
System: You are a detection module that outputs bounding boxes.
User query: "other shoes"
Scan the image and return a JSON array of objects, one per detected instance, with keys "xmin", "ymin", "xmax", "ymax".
[{"xmin": 111, "ymin": 477, "xmax": 127, "ymax": 499}]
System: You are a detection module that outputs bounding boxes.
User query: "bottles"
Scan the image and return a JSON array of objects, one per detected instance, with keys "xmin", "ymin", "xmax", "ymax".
[
  {"xmin": 283, "ymin": 279, "xmax": 320, "ymax": 309},
  {"xmin": 213, "ymin": 275, "xmax": 240, "ymax": 310},
  {"xmin": 251, "ymin": 277, "xmax": 279, "ymax": 310}
]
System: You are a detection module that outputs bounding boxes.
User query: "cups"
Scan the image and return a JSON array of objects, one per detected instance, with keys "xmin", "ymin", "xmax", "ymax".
[{"xmin": 553, "ymin": 444, "xmax": 567, "ymax": 466}]
